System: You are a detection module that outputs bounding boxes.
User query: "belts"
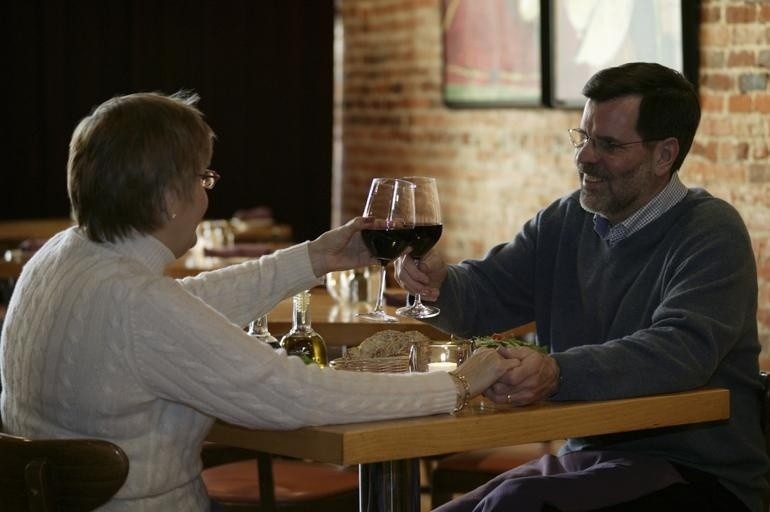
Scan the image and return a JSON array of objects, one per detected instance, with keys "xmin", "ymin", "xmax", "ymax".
[{"xmin": 330, "ymin": 356, "xmax": 414, "ymax": 374}]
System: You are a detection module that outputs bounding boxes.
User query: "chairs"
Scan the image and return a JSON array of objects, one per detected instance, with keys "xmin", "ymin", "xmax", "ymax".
[{"xmin": 2, "ymin": 424, "xmax": 132, "ymax": 509}]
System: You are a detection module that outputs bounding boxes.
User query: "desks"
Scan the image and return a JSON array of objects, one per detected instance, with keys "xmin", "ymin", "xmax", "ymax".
[
  {"xmin": 3, "ymin": 223, "xmax": 456, "ymax": 348},
  {"xmin": 200, "ymin": 380, "xmax": 730, "ymax": 510}
]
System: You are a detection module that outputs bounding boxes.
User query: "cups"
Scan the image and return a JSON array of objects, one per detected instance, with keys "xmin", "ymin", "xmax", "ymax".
[
  {"xmin": 326, "ymin": 271, "xmax": 358, "ymax": 302},
  {"xmin": 427, "ymin": 344, "xmax": 458, "ymax": 374}
]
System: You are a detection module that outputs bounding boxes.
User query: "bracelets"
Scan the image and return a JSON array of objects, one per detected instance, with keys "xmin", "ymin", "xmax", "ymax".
[{"xmin": 449, "ymin": 370, "xmax": 470, "ymax": 415}]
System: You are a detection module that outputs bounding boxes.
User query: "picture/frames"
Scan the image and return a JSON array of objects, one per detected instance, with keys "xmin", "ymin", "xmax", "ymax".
[
  {"xmin": 439, "ymin": 0, "xmax": 546, "ymax": 108},
  {"xmin": 545, "ymin": 0, "xmax": 699, "ymax": 109}
]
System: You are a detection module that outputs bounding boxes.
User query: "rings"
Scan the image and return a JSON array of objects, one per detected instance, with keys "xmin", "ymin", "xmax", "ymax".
[{"xmin": 506, "ymin": 393, "xmax": 513, "ymax": 407}]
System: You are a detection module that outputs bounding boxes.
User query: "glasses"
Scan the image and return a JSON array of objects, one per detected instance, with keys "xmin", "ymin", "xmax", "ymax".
[
  {"xmin": 569, "ymin": 128, "xmax": 663, "ymax": 155},
  {"xmin": 196, "ymin": 169, "xmax": 221, "ymax": 189}
]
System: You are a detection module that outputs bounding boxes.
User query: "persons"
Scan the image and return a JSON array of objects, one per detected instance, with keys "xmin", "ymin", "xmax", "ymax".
[
  {"xmin": 391, "ymin": 59, "xmax": 770, "ymax": 511},
  {"xmin": 2, "ymin": 83, "xmax": 521, "ymax": 510}
]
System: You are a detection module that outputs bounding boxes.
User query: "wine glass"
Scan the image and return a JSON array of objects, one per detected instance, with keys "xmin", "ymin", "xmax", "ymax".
[
  {"xmin": 353, "ymin": 178, "xmax": 415, "ymax": 326},
  {"xmin": 394, "ymin": 179, "xmax": 445, "ymax": 321}
]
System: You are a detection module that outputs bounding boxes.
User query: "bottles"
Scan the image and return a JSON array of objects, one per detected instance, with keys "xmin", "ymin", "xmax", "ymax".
[
  {"xmin": 280, "ymin": 290, "xmax": 329, "ymax": 371},
  {"xmin": 246, "ymin": 314, "xmax": 279, "ymax": 350}
]
